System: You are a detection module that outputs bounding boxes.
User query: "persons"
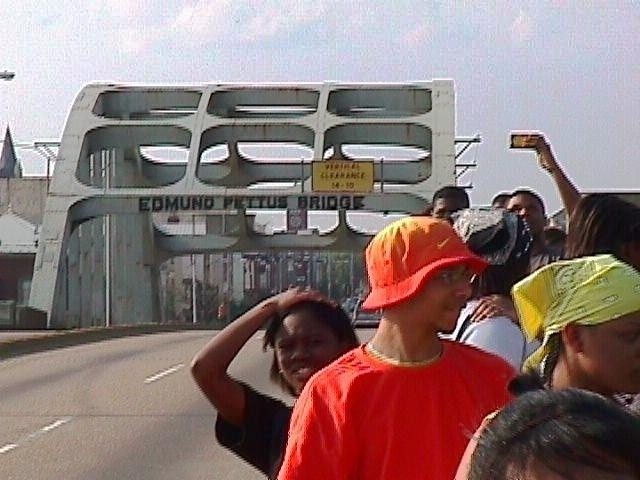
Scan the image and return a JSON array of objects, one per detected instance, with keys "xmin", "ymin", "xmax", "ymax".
[
  {"xmin": 509, "ymin": 254, "xmax": 640, "ymax": 414},
  {"xmin": 430, "ymin": 131, "xmax": 583, "ymax": 377},
  {"xmin": 566, "ymin": 190, "xmax": 640, "ymax": 275},
  {"xmin": 443, "ymin": 386, "xmax": 640, "ymax": 480},
  {"xmin": 189, "ymin": 286, "xmax": 364, "ymax": 480},
  {"xmin": 275, "ymin": 216, "xmax": 522, "ymax": 480}
]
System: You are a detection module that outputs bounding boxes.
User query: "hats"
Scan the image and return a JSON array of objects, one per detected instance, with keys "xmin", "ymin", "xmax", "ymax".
[{"xmin": 358, "ymin": 206, "xmax": 534, "ymax": 312}]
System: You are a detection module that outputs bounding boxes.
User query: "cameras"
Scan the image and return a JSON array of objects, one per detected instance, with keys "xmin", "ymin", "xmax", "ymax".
[{"xmin": 510, "ymin": 134, "xmax": 541, "ymax": 148}]
